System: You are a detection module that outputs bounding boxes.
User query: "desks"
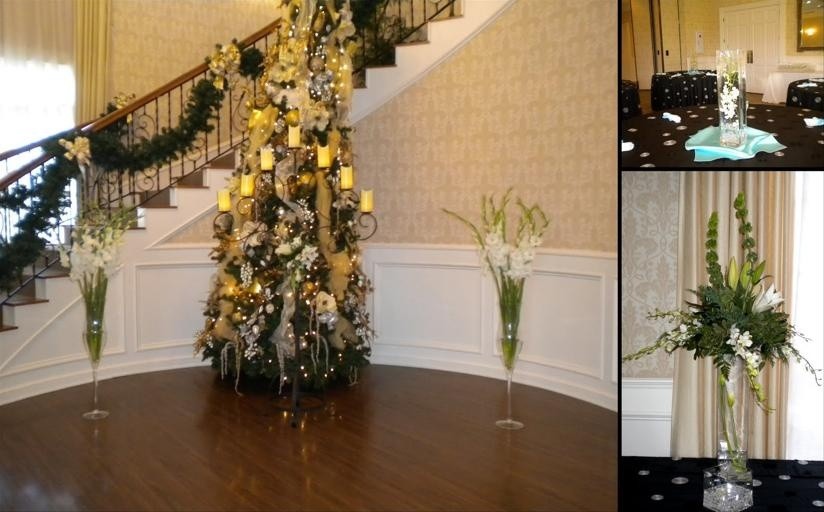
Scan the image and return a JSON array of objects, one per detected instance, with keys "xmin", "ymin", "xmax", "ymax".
[
  {"xmin": 621, "ymin": 80, "xmax": 642, "ymax": 121},
  {"xmin": 650, "ymin": 70, "xmax": 718, "ymax": 112},
  {"xmin": 620, "ymin": 104, "xmax": 824, "ymax": 168},
  {"xmin": 785, "ymin": 78, "xmax": 824, "ymax": 113},
  {"xmin": 620, "ymin": 454, "xmax": 823, "ymax": 511}
]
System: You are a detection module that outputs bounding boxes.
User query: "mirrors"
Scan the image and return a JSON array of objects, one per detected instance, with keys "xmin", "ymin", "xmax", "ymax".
[{"xmin": 795, "ymin": 0, "xmax": 823, "ymax": 52}]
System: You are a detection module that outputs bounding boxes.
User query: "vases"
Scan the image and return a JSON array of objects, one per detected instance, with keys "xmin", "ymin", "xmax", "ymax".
[{"xmin": 715, "ymin": 350, "xmax": 753, "ymax": 484}]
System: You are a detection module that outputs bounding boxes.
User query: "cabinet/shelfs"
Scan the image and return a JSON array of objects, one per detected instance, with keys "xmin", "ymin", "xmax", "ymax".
[{"xmin": 621, "ymin": 379, "xmax": 672, "ymax": 457}]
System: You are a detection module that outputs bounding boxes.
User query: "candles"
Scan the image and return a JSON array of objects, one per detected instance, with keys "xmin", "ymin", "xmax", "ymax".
[
  {"xmin": 239, "ymin": 173, "xmax": 254, "ymax": 197},
  {"xmin": 215, "ymin": 189, "xmax": 232, "ymax": 212},
  {"xmin": 316, "ymin": 144, "xmax": 330, "ymax": 168},
  {"xmin": 359, "ymin": 189, "xmax": 374, "ymax": 213},
  {"xmin": 260, "ymin": 147, "xmax": 273, "ymax": 171},
  {"xmin": 340, "ymin": 165, "xmax": 353, "ymax": 190},
  {"xmin": 287, "ymin": 124, "xmax": 301, "ymax": 148}
]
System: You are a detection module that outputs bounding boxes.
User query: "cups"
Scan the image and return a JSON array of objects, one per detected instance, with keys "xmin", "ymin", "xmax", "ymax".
[{"xmin": 703, "ymin": 461, "xmax": 754, "ymax": 512}]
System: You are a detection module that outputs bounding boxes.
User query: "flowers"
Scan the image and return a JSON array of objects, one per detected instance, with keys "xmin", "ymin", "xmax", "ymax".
[
  {"xmin": 719, "ymin": 55, "xmax": 741, "ymax": 120},
  {"xmin": 620, "ymin": 189, "xmax": 823, "ymax": 474},
  {"xmin": 438, "ymin": 187, "xmax": 551, "ymax": 369},
  {"xmin": 53, "ymin": 199, "xmax": 142, "ymax": 362}
]
